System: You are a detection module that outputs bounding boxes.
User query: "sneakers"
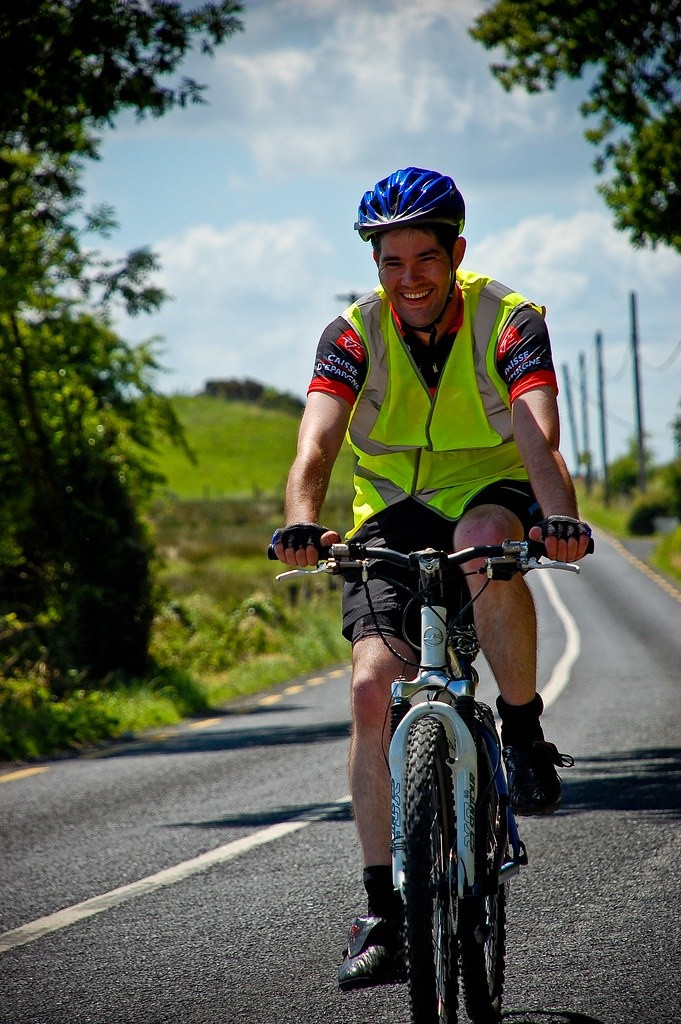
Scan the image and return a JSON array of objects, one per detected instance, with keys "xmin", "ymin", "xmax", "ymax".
[
  {"xmin": 336, "ymin": 916, "xmax": 404, "ymax": 991},
  {"xmin": 499, "ymin": 723, "xmax": 574, "ymax": 818}
]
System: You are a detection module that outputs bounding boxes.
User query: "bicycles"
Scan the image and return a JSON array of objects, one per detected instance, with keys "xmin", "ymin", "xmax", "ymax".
[{"xmin": 267, "ymin": 538, "xmax": 596, "ymax": 1024}]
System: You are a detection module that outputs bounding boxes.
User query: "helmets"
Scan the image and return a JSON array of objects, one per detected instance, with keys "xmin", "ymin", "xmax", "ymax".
[{"xmin": 353, "ymin": 167, "xmax": 467, "ymax": 241}]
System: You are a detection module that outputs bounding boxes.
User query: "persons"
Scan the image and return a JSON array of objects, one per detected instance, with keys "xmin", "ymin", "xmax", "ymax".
[{"xmin": 272, "ymin": 168, "xmax": 591, "ymax": 988}]
